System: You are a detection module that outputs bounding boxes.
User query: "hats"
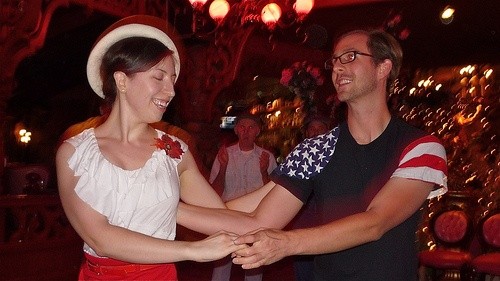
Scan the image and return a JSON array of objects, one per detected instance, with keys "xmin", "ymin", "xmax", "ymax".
[{"xmin": 86, "ymin": 14, "xmax": 183, "ymax": 100}]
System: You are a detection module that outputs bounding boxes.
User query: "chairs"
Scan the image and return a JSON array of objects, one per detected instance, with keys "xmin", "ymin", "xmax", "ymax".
[{"xmin": 417, "ymin": 206, "xmax": 500, "ymax": 281}]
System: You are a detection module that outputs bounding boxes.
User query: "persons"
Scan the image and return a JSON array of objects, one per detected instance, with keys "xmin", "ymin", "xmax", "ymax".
[
  {"xmin": 54, "ymin": 23, "xmax": 276, "ymax": 281},
  {"xmin": 208, "ymin": 113, "xmax": 278, "ymax": 281},
  {"xmin": 176, "ymin": 23, "xmax": 449, "ymax": 281}
]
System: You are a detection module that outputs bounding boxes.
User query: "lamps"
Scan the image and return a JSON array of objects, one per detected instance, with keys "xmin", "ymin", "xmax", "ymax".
[{"xmin": 186, "ymin": 0, "xmax": 313, "ymax": 43}]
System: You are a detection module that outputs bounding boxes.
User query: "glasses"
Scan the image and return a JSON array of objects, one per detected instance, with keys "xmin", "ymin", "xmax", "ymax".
[{"xmin": 324, "ymin": 50, "xmax": 377, "ymax": 71}]
